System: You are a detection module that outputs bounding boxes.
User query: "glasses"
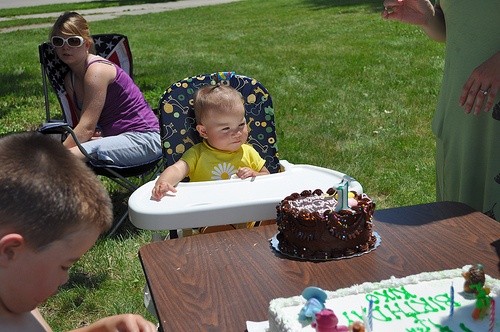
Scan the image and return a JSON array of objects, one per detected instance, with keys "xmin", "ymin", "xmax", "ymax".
[{"xmin": 50, "ymin": 36, "xmax": 87, "ymax": 48}]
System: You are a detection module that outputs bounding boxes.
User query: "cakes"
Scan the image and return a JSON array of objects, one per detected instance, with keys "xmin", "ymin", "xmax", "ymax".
[
  {"xmin": 269, "ymin": 263, "xmax": 500, "ymax": 332},
  {"xmin": 275, "ymin": 188, "xmax": 376, "ymax": 259}
]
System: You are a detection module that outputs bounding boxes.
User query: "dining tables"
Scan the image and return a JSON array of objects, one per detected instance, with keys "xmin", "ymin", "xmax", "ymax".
[{"xmin": 137, "ymin": 201, "xmax": 500, "ymax": 332}]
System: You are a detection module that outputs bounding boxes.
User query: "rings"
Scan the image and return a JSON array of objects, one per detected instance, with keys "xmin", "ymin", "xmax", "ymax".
[{"xmin": 480, "ymin": 90, "xmax": 488, "ymax": 96}]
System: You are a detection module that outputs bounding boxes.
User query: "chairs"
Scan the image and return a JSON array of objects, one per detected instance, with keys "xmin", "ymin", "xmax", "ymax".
[
  {"xmin": 36, "ymin": 33, "xmax": 167, "ymax": 239},
  {"xmin": 158, "ymin": 71, "xmax": 281, "ymax": 238}
]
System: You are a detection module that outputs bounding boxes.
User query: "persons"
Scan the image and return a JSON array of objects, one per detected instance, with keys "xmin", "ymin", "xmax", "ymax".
[
  {"xmin": 0, "ymin": 131, "xmax": 158, "ymax": 332},
  {"xmin": 49, "ymin": 12, "xmax": 162, "ymax": 169},
  {"xmin": 381, "ymin": 0, "xmax": 500, "ymax": 223},
  {"xmin": 151, "ymin": 85, "xmax": 276, "ymax": 234}
]
